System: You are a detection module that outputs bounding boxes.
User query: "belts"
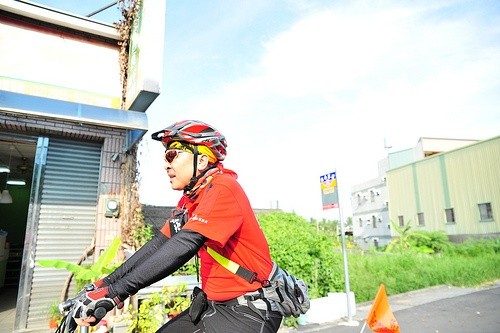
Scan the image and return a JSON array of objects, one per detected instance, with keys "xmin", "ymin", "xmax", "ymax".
[{"xmin": 215, "ymin": 295, "xmax": 258, "ymax": 307}]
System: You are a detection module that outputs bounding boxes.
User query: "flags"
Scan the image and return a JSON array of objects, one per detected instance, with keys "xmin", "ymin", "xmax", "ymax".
[{"xmin": 363, "ymin": 283, "xmax": 400, "ymax": 333}]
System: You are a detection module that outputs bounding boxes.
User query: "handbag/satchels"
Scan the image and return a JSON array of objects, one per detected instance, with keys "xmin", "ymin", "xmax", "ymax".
[
  {"xmin": 188, "ymin": 287, "xmax": 206, "ymax": 322},
  {"xmin": 261, "ymin": 261, "xmax": 310, "ymax": 318}
]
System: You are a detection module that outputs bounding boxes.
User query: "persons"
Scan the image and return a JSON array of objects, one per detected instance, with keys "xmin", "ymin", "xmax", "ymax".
[{"xmin": 71, "ymin": 119, "xmax": 310, "ymax": 333}]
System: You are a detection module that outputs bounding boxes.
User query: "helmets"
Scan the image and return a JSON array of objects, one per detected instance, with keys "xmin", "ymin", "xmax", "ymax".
[{"xmin": 151, "ymin": 120, "xmax": 228, "ymax": 161}]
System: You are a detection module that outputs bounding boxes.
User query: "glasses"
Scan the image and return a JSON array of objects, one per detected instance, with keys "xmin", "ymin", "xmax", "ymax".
[{"xmin": 164, "ymin": 150, "xmax": 184, "ymax": 164}]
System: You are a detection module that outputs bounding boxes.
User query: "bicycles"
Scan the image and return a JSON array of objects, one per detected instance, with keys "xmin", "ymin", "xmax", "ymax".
[{"xmin": 52, "ymin": 300, "xmax": 108, "ymax": 333}]
